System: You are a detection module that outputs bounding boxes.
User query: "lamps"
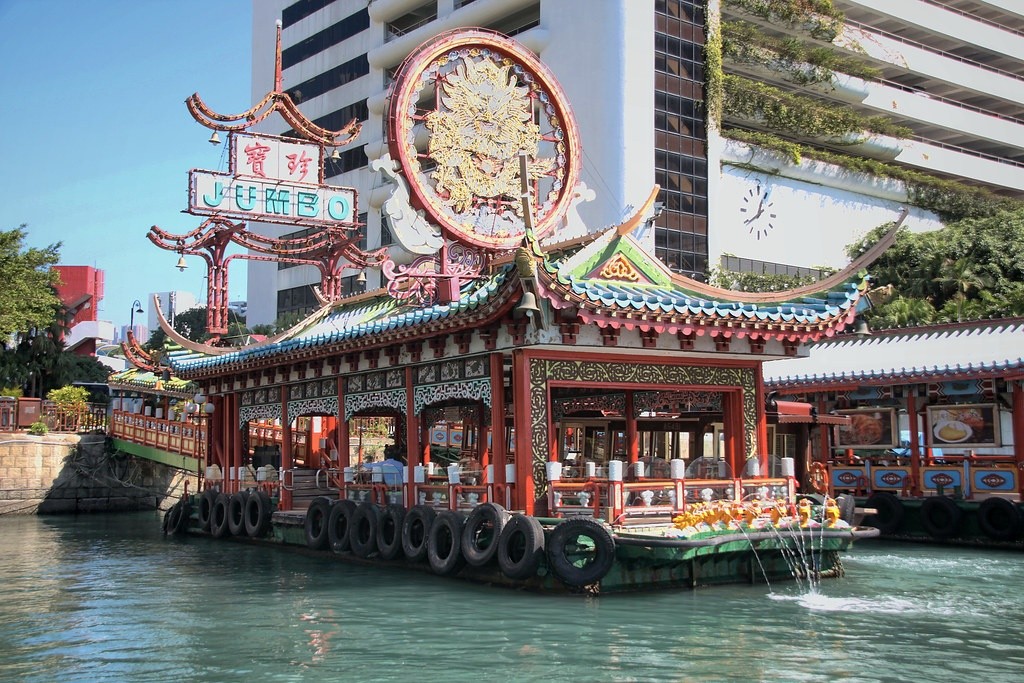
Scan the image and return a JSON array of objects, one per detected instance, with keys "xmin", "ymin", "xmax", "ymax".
[
  {"xmin": 516, "ymin": 291, "xmax": 540, "ymax": 319},
  {"xmin": 159, "ymin": 368, "xmax": 173, "ymax": 384},
  {"xmin": 852, "ymin": 316, "xmax": 872, "ymax": 339},
  {"xmin": 174, "ymin": 253, "xmax": 189, "ymax": 272},
  {"xmin": 329, "ymin": 146, "xmax": 342, "ymax": 164},
  {"xmin": 207, "ymin": 128, "xmax": 222, "ymax": 146},
  {"xmin": 355, "ymin": 269, "xmax": 368, "ymax": 286}
]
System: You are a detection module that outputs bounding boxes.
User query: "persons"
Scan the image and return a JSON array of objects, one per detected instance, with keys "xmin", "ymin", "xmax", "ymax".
[{"xmin": 359, "ymin": 447, "xmax": 406, "ymax": 483}]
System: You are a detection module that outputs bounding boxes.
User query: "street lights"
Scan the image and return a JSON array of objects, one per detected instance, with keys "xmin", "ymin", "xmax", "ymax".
[{"xmin": 130, "ymin": 300, "xmax": 144, "ymax": 331}]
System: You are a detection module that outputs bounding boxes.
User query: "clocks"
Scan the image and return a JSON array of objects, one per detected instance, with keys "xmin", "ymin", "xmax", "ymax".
[{"xmin": 740, "ymin": 185, "xmax": 777, "ymax": 240}]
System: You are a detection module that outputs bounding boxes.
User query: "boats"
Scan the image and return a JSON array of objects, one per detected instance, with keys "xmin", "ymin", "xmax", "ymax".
[{"xmin": 118, "ymin": 19, "xmax": 909, "ymax": 596}]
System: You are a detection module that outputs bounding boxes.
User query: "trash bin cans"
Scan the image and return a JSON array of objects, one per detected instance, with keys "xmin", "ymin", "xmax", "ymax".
[
  {"xmin": 0, "ymin": 395, "xmax": 15, "ymax": 426},
  {"xmin": 16, "ymin": 397, "xmax": 41, "ymax": 430},
  {"xmin": 42, "ymin": 400, "xmax": 58, "ymax": 428}
]
